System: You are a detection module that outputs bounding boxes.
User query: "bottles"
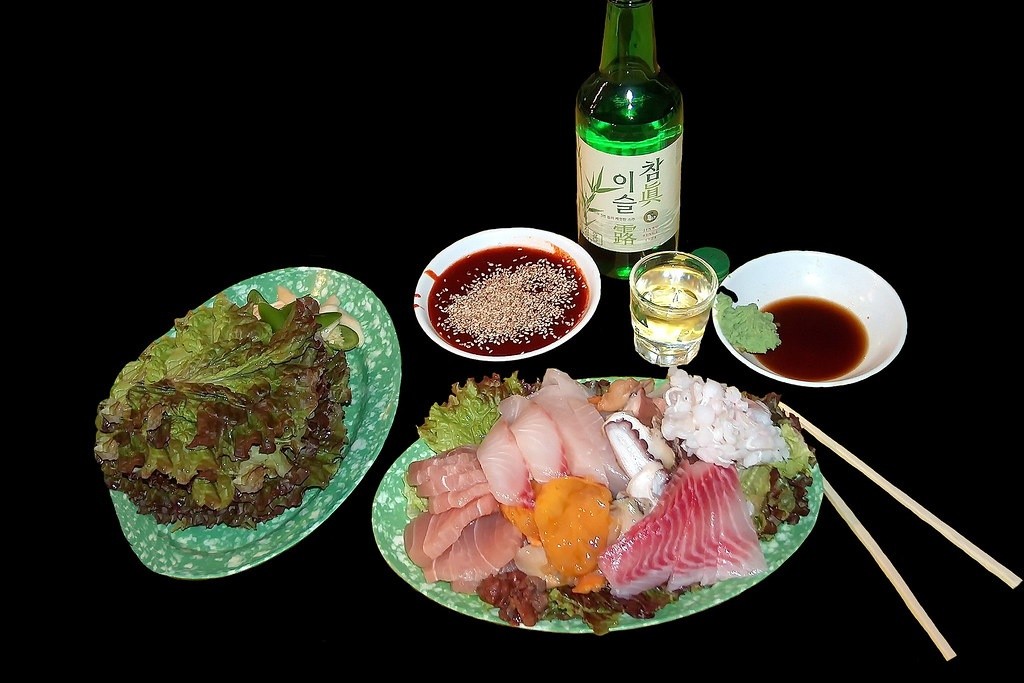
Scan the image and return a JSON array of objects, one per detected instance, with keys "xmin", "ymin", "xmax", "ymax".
[{"xmin": 574, "ymin": 0, "xmax": 685, "ymax": 280}]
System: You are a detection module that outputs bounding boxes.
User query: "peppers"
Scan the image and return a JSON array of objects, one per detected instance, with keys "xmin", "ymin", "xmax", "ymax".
[{"xmin": 246, "ymin": 289, "xmax": 360, "ymax": 352}]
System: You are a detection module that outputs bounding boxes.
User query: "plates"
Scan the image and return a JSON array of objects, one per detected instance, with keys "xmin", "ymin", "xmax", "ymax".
[
  {"xmin": 109, "ymin": 266, "xmax": 402, "ymax": 580},
  {"xmin": 413, "ymin": 228, "xmax": 602, "ymax": 361},
  {"xmin": 712, "ymin": 250, "xmax": 907, "ymax": 387},
  {"xmin": 372, "ymin": 377, "xmax": 823, "ymax": 633}
]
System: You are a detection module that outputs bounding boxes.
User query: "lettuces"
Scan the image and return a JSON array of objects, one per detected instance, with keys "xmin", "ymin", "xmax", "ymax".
[
  {"xmin": 94, "ymin": 292, "xmax": 352, "ymax": 531},
  {"xmin": 401, "ymin": 371, "xmax": 820, "ymax": 638}
]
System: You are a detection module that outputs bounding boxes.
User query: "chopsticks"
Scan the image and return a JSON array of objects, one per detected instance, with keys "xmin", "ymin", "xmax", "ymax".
[{"xmin": 777, "ymin": 401, "xmax": 1022, "ymax": 662}]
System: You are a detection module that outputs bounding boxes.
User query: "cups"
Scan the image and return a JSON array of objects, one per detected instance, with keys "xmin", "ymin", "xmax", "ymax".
[{"xmin": 629, "ymin": 251, "xmax": 718, "ymax": 367}]
